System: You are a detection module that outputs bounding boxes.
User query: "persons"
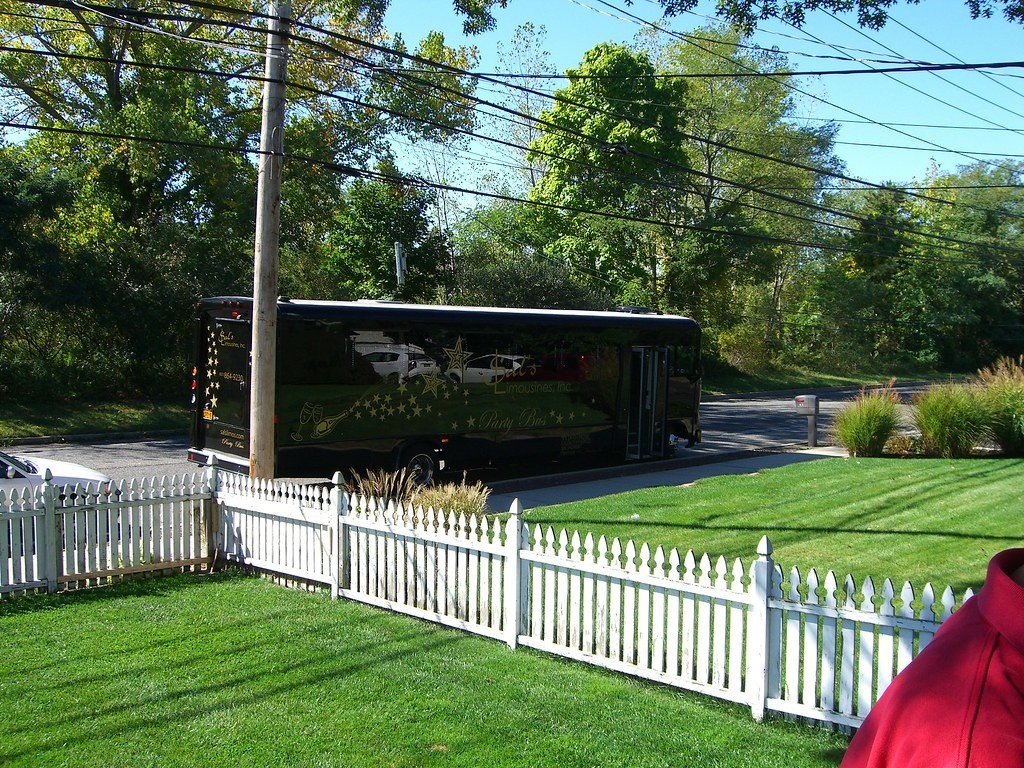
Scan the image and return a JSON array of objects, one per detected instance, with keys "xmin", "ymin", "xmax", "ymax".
[{"xmin": 841, "ymin": 549, "xmax": 1024, "ymax": 767}]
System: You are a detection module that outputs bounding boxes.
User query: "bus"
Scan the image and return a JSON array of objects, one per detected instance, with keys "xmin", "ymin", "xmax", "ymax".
[{"xmin": 187, "ymin": 297, "xmax": 703, "ymax": 491}]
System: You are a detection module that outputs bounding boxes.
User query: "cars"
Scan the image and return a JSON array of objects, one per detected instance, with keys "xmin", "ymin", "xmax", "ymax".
[{"xmin": 0, "ymin": 450, "xmax": 119, "ymax": 535}]
